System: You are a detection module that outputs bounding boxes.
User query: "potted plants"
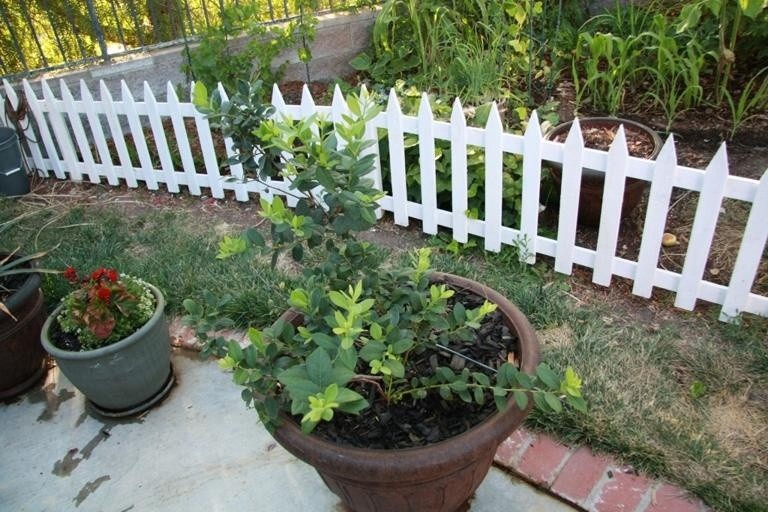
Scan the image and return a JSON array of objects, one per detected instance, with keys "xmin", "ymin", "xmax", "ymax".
[
  {"xmin": 182, "ymin": 78, "xmax": 588, "ymax": 512},
  {"xmin": 0, "ymin": 241, "xmax": 62, "ymax": 399},
  {"xmin": 41, "ymin": 280, "xmax": 177, "ymax": 418}
]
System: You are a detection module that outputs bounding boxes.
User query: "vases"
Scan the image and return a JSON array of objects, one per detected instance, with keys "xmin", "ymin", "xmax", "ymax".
[{"xmin": 547, "ymin": 116, "xmax": 664, "ymax": 216}]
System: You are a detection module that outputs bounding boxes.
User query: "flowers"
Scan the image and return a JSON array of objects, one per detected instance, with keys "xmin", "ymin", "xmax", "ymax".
[{"xmin": 56, "ymin": 266, "xmax": 157, "ymax": 346}]
